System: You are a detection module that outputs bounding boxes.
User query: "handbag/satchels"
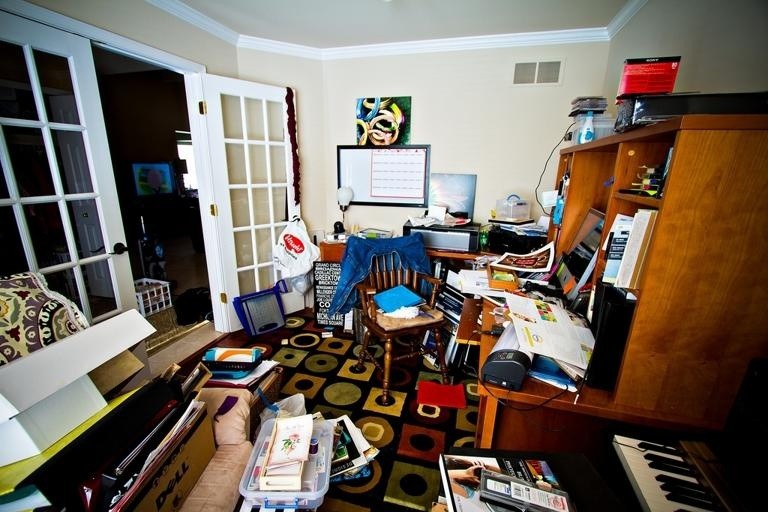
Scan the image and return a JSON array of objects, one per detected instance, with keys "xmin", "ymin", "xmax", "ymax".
[{"xmin": 274, "ymin": 217, "xmax": 321, "ymax": 281}]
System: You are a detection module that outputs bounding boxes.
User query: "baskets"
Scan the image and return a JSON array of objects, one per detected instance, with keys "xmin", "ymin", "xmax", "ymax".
[{"xmin": 133, "ymin": 278, "xmax": 173, "ymax": 318}]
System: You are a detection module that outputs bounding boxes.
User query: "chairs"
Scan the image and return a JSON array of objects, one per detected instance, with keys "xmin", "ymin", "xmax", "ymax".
[{"xmin": 346, "ymin": 233, "xmax": 446, "ymax": 404}]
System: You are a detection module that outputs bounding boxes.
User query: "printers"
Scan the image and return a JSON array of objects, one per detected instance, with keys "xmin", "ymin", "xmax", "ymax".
[{"xmin": 403, "ymin": 218, "xmax": 481, "ymax": 253}]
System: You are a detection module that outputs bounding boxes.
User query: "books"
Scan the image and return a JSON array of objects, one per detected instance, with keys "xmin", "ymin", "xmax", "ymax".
[
  {"xmin": 437, "ymin": 452, "xmax": 570, "ymax": 512},
  {"xmin": 135, "ymin": 282, "xmax": 170, "ymax": 315},
  {"xmin": 326, "ymin": 227, "xmax": 397, "ymax": 244},
  {"xmin": 204, "ymin": 360, "xmax": 280, "ymax": 397},
  {"xmin": 0, "ymin": 360, "xmax": 213, "ymax": 512},
  {"xmin": 246, "ymin": 408, "xmax": 380, "ymax": 506},
  {"xmin": 601, "ymin": 208, "xmax": 658, "ymax": 301},
  {"xmin": 568, "ymin": 95, "xmax": 609, "ymax": 117},
  {"xmin": 458, "ymin": 216, "xmax": 604, "ymax": 392},
  {"xmin": 374, "ymin": 284, "xmax": 427, "ymax": 314}
]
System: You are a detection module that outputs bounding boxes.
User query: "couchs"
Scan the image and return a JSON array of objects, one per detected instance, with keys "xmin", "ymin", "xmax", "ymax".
[{"xmin": 170, "ymin": 387, "xmax": 252, "ymax": 510}]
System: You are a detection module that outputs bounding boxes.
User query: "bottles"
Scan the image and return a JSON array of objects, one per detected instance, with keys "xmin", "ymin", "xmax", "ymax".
[
  {"xmin": 479, "ymin": 226, "xmax": 488, "ymax": 252},
  {"xmin": 578, "ymin": 110, "xmax": 594, "ymax": 145}
]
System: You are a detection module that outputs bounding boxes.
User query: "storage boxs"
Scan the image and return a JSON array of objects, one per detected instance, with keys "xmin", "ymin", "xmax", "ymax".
[{"xmin": 1, "ymin": 309, "xmax": 217, "ymax": 512}]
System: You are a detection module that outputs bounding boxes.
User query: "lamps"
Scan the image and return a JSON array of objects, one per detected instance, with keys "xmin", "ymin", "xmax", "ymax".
[{"xmin": 335, "ymin": 187, "xmax": 353, "ymax": 230}]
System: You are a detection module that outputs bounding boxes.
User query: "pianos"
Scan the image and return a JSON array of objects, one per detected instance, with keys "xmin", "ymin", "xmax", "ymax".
[{"xmin": 613, "ymin": 434, "xmax": 746, "ymax": 512}]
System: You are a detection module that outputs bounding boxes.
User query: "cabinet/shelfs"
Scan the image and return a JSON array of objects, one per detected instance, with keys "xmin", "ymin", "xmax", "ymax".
[{"xmin": 469, "ymin": 115, "xmax": 768, "ymax": 444}]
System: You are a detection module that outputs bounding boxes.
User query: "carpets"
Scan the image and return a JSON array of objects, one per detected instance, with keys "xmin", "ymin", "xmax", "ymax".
[{"xmin": 168, "ymin": 314, "xmax": 469, "ymax": 509}]
